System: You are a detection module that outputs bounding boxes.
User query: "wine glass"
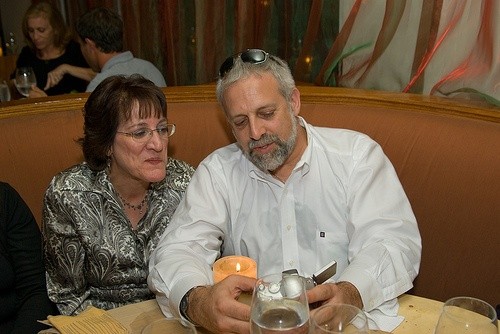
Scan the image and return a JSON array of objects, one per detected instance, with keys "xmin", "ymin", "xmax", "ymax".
[{"xmin": 16, "ymin": 67, "xmax": 37, "ymax": 98}]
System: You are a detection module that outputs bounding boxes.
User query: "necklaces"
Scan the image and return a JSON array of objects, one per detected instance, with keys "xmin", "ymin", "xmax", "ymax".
[{"xmin": 117, "ymin": 192, "xmax": 150, "ymax": 214}]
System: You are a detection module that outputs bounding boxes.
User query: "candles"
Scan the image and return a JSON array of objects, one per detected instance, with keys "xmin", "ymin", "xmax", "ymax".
[{"xmin": 213, "ymin": 256, "xmax": 257, "ymax": 285}]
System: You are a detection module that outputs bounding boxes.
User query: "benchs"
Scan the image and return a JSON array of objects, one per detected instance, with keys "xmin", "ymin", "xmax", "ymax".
[{"xmin": 0, "ymin": 85, "xmax": 500, "ymax": 320}]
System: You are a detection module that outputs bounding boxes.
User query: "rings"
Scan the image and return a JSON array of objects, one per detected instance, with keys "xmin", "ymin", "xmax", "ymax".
[{"xmin": 323, "ymin": 323, "xmax": 330, "ymax": 333}]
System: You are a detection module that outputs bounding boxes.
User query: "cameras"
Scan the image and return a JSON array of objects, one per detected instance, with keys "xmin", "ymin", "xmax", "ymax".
[{"xmin": 279, "ymin": 261, "xmax": 337, "ymax": 297}]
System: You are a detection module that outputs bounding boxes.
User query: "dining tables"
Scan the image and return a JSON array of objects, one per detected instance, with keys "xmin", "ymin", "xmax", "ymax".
[{"xmin": 38, "ymin": 295, "xmax": 500, "ymax": 334}]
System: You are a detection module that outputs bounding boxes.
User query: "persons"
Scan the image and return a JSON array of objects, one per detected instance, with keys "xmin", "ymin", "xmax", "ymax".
[
  {"xmin": 10, "ymin": 0, "xmax": 98, "ymax": 95},
  {"xmin": 42, "ymin": 75, "xmax": 195, "ymax": 318},
  {"xmin": 76, "ymin": 8, "xmax": 168, "ymax": 92},
  {"xmin": 147, "ymin": 49, "xmax": 422, "ymax": 333},
  {"xmin": 0, "ymin": 179, "xmax": 59, "ymax": 333}
]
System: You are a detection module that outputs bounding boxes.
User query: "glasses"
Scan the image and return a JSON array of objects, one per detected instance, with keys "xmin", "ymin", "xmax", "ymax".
[
  {"xmin": 116, "ymin": 122, "xmax": 176, "ymax": 144},
  {"xmin": 219, "ymin": 49, "xmax": 283, "ymax": 80}
]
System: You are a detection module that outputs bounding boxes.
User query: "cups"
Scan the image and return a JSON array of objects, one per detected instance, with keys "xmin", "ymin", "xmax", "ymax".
[
  {"xmin": 312, "ymin": 303, "xmax": 370, "ymax": 334},
  {"xmin": 434, "ymin": 296, "xmax": 498, "ymax": 334},
  {"xmin": 213, "ymin": 255, "xmax": 257, "ymax": 304},
  {"xmin": 141, "ymin": 317, "xmax": 199, "ymax": 334},
  {"xmin": 250, "ymin": 273, "xmax": 311, "ymax": 334}
]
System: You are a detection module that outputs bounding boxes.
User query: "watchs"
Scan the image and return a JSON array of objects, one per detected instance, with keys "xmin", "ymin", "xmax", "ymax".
[{"xmin": 180, "ymin": 286, "xmax": 206, "ymax": 326}]
